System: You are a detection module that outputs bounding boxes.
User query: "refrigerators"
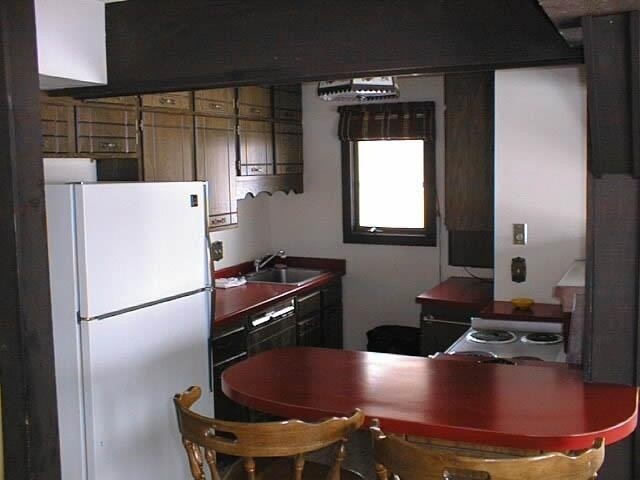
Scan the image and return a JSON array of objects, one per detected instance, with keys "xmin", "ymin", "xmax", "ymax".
[{"xmin": 42, "ymin": 179, "xmax": 217, "ymax": 478}]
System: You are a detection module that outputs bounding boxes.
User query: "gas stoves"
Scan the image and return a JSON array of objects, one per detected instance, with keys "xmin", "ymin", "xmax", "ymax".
[{"xmin": 435, "ymin": 324, "xmax": 566, "ymax": 363}]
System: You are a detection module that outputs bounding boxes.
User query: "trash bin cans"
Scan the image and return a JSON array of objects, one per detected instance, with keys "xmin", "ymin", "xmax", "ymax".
[{"xmin": 366, "ymin": 325, "xmax": 421, "ymax": 356}]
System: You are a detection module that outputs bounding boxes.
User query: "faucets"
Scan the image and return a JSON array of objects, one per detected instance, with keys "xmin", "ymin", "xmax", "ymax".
[{"xmin": 255, "ymin": 250, "xmax": 287, "ymax": 272}]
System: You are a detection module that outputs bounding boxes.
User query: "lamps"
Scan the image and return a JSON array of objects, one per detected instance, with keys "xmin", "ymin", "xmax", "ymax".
[{"xmin": 314, "ymin": 76, "xmax": 405, "ymax": 103}]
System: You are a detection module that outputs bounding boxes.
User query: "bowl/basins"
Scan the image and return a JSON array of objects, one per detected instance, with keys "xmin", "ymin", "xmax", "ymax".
[{"xmin": 512, "ymin": 297, "xmax": 534, "ymax": 312}]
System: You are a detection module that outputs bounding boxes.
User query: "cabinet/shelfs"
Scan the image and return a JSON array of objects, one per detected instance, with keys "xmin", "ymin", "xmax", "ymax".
[
  {"xmin": 414, "ymin": 272, "xmax": 494, "ymax": 354},
  {"xmin": 294, "ymin": 281, "xmax": 326, "ymax": 349},
  {"xmin": 99, "ymin": 110, "xmax": 197, "ymax": 182},
  {"xmin": 322, "ymin": 272, "xmax": 344, "ymax": 349},
  {"xmin": 191, "ymin": 111, "xmax": 243, "ymax": 231},
  {"xmin": 139, "ymin": 78, "xmax": 195, "ymax": 117},
  {"xmin": 210, "ymin": 318, "xmax": 255, "ymax": 467},
  {"xmin": 272, "ymin": 80, "xmax": 304, "ymax": 128},
  {"xmin": 237, "ymin": 82, "xmax": 276, "ymax": 123},
  {"xmin": 229, "ymin": 115, "xmax": 308, "ymax": 199},
  {"xmin": 76, "ymin": 94, "xmax": 142, "ymax": 161},
  {"xmin": 490, "ymin": 58, "xmax": 588, "ymax": 309},
  {"xmin": 34, "ymin": 1, "xmax": 111, "ymax": 94},
  {"xmin": 39, "ymin": 91, "xmax": 78, "ymax": 161},
  {"xmin": 193, "ymin": 84, "xmax": 238, "ymax": 121}
]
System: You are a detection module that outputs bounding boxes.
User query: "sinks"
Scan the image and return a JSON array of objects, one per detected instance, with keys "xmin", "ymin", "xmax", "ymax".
[{"xmin": 238, "ymin": 266, "xmax": 330, "ymax": 287}]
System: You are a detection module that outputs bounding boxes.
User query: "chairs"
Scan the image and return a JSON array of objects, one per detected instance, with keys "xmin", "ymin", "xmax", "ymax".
[
  {"xmin": 355, "ymin": 420, "xmax": 611, "ymax": 478},
  {"xmin": 171, "ymin": 378, "xmax": 365, "ymax": 478}
]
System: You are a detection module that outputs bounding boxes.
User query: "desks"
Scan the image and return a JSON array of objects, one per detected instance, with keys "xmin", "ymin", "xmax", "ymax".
[{"xmin": 217, "ymin": 342, "xmax": 638, "ymax": 480}]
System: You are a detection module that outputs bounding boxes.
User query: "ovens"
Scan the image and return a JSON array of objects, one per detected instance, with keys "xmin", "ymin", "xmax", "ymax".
[{"xmin": 248, "ymin": 314, "xmax": 297, "ymax": 423}]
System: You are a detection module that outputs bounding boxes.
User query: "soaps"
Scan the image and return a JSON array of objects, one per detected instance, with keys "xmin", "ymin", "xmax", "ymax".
[{"xmin": 274, "ymin": 264, "xmax": 287, "ymax": 269}]
